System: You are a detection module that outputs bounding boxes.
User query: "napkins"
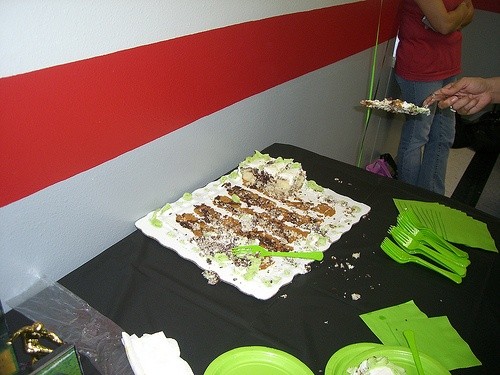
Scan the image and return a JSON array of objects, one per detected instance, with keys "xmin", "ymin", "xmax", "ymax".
[{"xmin": 119, "ymin": 331, "xmax": 194, "ymax": 374}]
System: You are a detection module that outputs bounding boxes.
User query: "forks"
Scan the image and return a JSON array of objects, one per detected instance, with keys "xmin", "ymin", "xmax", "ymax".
[
  {"xmin": 380, "ymin": 211, "xmax": 471, "ymax": 283},
  {"xmin": 231, "ymin": 245, "xmax": 324, "ymax": 261}
]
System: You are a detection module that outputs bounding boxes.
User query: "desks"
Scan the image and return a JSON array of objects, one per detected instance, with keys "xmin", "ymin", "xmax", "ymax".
[{"xmin": 399, "ymin": 8, "xmax": 500, "ymax": 209}]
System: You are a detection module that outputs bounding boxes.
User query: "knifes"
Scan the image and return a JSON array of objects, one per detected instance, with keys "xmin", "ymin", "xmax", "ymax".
[{"xmin": 360, "ymin": 94, "xmax": 461, "ymax": 115}]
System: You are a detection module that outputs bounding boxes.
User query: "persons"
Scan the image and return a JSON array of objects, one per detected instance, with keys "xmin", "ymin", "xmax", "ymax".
[
  {"xmin": 422, "ymin": 76, "xmax": 500, "ymax": 115},
  {"xmin": 393, "ymin": 0, "xmax": 474, "ymax": 196}
]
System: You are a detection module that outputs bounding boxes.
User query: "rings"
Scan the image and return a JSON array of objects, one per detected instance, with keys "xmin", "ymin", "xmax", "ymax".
[{"xmin": 449, "ymin": 103, "xmax": 458, "ymax": 114}]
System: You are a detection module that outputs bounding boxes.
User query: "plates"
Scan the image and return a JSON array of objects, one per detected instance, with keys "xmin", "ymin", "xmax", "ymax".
[
  {"xmin": 342, "ymin": 345, "xmax": 454, "ymax": 375},
  {"xmin": 325, "ymin": 342, "xmax": 386, "ymax": 375},
  {"xmin": 203, "ymin": 345, "xmax": 315, "ymax": 375}
]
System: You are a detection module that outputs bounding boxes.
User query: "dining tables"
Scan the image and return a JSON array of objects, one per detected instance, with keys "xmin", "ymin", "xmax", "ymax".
[{"xmin": 0, "ymin": 143, "xmax": 500, "ymax": 375}]
